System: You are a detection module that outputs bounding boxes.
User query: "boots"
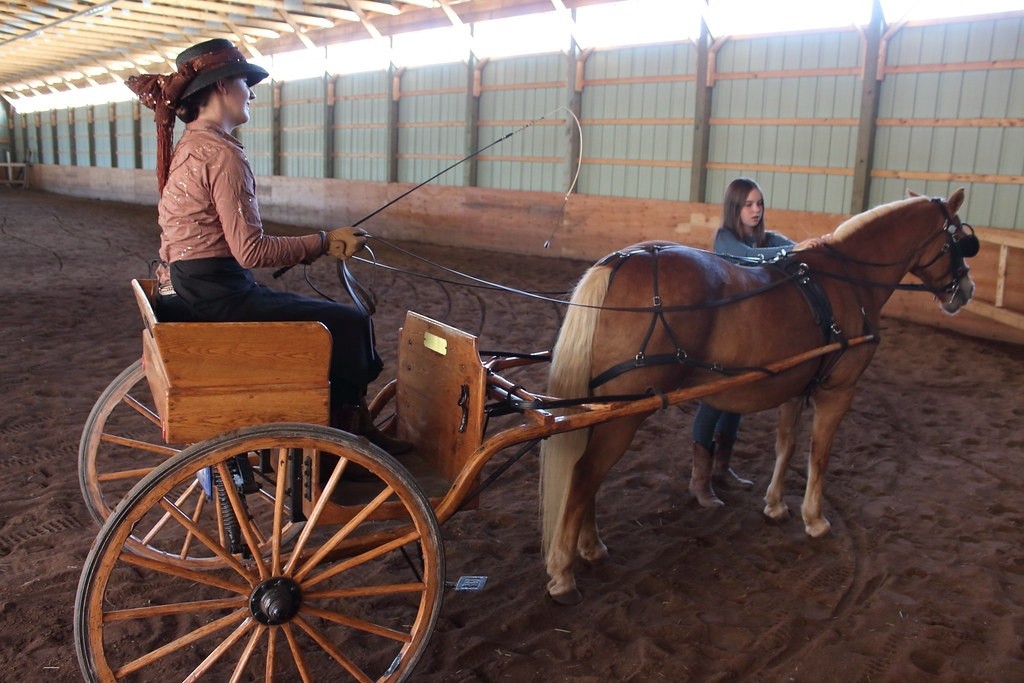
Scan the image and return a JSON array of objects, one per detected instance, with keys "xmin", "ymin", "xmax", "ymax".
[
  {"xmin": 711, "ymin": 431, "xmax": 754, "ymax": 489},
  {"xmin": 330, "ymin": 400, "xmax": 379, "ymax": 480},
  {"xmin": 689, "ymin": 442, "xmax": 725, "ymax": 507},
  {"xmin": 360, "ymin": 398, "xmax": 414, "ymax": 454}
]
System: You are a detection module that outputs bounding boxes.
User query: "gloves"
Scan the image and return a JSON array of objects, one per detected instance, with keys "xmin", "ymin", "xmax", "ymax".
[{"xmin": 320, "ymin": 227, "xmax": 368, "ymax": 259}]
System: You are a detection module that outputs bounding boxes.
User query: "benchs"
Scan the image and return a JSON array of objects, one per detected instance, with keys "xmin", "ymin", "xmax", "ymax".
[{"xmin": 130, "ymin": 260, "xmax": 335, "ymax": 523}]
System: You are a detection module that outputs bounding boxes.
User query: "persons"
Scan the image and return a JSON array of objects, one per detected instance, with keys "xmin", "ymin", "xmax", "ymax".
[
  {"xmin": 689, "ymin": 178, "xmax": 834, "ymax": 507},
  {"xmin": 157, "ymin": 39, "xmax": 416, "ymax": 480}
]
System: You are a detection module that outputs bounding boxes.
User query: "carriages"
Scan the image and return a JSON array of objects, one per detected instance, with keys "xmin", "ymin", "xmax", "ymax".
[{"xmin": 73, "ymin": 185, "xmax": 979, "ymax": 683}]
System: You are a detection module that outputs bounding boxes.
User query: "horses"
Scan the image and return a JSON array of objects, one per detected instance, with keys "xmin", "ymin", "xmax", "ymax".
[{"xmin": 538, "ymin": 187, "xmax": 975, "ymax": 604}]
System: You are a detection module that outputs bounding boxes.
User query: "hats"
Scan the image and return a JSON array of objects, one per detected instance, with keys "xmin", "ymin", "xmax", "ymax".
[{"xmin": 173, "ymin": 38, "xmax": 269, "ymax": 100}]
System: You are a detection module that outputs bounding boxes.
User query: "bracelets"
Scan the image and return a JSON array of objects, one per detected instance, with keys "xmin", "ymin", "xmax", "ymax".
[{"xmin": 319, "ymin": 231, "xmax": 327, "ymax": 255}]
